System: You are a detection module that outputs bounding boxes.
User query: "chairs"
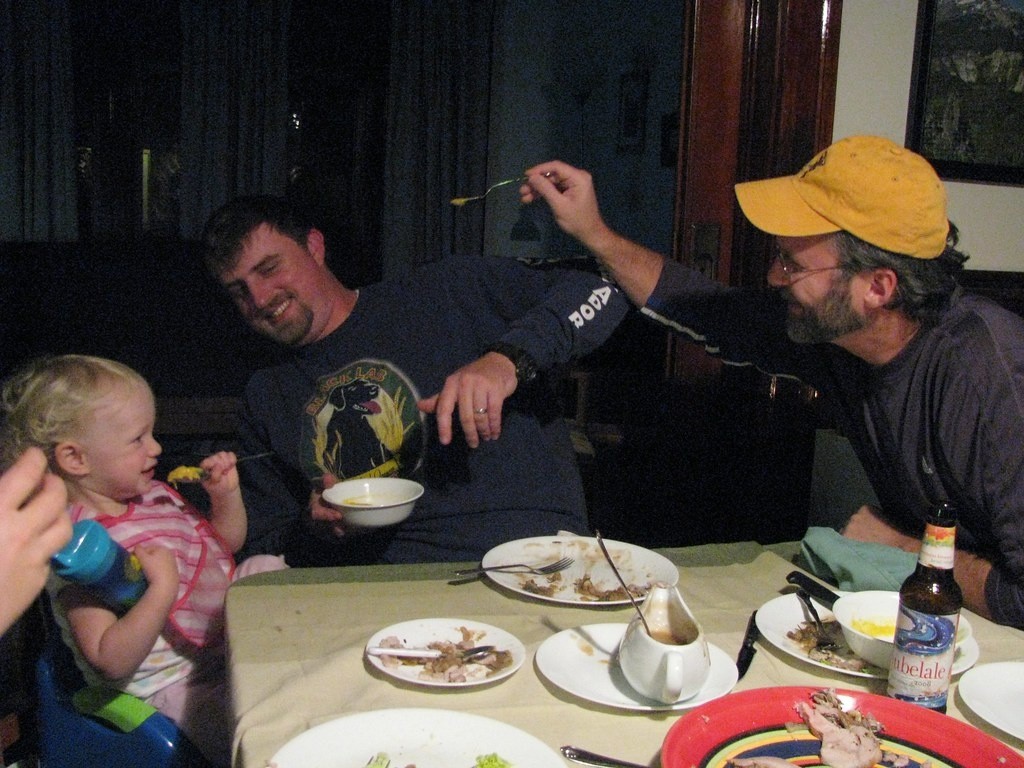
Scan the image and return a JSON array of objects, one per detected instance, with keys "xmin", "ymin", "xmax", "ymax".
[{"xmin": 36, "ymin": 641, "xmax": 213, "ymax": 768}]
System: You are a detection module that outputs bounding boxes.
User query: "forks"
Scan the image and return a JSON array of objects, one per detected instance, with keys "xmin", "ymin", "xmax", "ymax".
[
  {"xmin": 455, "ymin": 556, "xmax": 576, "ymax": 579},
  {"xmin": 796, "ymin": 591, "xmax": 841, "ymax": 651}
]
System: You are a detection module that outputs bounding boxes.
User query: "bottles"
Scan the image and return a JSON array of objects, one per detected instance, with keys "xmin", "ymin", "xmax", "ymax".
[
  {"xmin": 49, "ymin": 520, "xmax": 150, "ymax": 611},
  {"xmin": 888, "ymin": 502, "xmax": 961, "ymax": 715}
]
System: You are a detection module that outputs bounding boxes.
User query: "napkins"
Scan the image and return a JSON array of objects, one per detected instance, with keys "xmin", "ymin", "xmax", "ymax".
[{"xmin": 801, "ymin": 526, "xmax": 921, "ymax": 591}]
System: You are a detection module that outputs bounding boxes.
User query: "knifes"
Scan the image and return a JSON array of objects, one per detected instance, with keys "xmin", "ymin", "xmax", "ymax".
[{"xmin": 736, "ymin": 609, "xmax": 762, "ymax": 680}]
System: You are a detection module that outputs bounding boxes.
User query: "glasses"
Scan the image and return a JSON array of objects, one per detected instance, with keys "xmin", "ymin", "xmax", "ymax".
[{"xmin": 774, "ymin": 242, "xmax": 848, "ymax": 277}]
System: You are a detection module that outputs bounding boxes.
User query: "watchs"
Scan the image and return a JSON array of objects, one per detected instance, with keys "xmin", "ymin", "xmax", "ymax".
[{"xmin": 484, "ymin": 341, "xmax": 543, "ymax": 393}]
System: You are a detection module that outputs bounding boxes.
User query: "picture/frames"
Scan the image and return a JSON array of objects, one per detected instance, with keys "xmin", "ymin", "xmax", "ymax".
[{"xmin": 904, "ymin": 0, "xmax": 1024, "ymax": 186}]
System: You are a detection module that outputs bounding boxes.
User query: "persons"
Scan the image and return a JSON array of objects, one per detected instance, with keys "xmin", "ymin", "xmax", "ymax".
[
  {"xmin": 519, "ymin": 136, "xmax": 1024, "ymax": 632},
  {"xmin": 0, "ymin": 445, "xmax": 73, "ymax": 637},
  {"xmin": 202, "ymin": 196, "xmax": 634, "ymax": 566},
  {"xmin": 0, "ymin": 353, "xmax": 287, "ymax": 768}
]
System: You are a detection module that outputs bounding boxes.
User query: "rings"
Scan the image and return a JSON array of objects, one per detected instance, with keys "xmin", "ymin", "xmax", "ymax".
[{"xmin": 475, "ymin": 407, "xmax": 487, "ymax": 414}]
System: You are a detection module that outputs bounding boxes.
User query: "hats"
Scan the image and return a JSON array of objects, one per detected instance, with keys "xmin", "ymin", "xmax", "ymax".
[{"xmin": 734, "ymin": 136, "xmax": 950, "ymax": 260}]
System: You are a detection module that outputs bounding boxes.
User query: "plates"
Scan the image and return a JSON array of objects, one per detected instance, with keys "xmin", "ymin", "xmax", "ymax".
[
  {"xmin": 482, "ymin": 535, "xmax": 680, "ymax": 606},
  {"xmin": 660, "ymin": 685, "xmax": 1024, "ymax": 768},
  {"xmin": 365, "ymin": 618, "xmax": 527, "ymax": 686},
  {"xmin": 535, "ymin": 621, "xmax": 738, "ymax": 711},
  {"xmin": 958, "ymin": 660, "xmax": 1024, "ymax": 742},
  {"xmin": 754, "ymin": 591, "xmax": 979, "ymax": 681},
  {"xmin": 265, "ymin": 707, "xmax": 572, "ymax": 767}
]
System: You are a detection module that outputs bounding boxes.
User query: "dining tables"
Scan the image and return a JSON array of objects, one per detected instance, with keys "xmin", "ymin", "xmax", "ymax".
[{"xmin": 224, "ymin": 539, "xmax": 1024, "ymax": 767}]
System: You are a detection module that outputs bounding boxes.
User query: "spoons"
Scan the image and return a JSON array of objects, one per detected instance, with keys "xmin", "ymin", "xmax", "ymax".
[
  {"xmin": 169, "ymin": 450, "xmax": 275, "ymax": 485},
  {"xmin": 451, "ymin": 171, "xmax": 554, "ymax": 206},
  {"xmin": 369, "ymin": 645, "xmax": 495, "ymax": 661}
]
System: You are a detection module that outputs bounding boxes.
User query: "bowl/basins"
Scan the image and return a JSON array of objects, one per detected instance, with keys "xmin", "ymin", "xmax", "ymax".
[
  {"xmin": 832, "ymin": 589, "xmax": 974, "ymax": 670},
  {"xmin": 321, "ymin": 477, "xmax": 424, "ymax": 527}
]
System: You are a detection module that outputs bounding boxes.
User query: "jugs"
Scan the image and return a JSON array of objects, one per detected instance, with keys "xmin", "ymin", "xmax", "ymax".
[{"xmin": 619, "ymin": 581, "xmax": 711, "ymax": 705}]
